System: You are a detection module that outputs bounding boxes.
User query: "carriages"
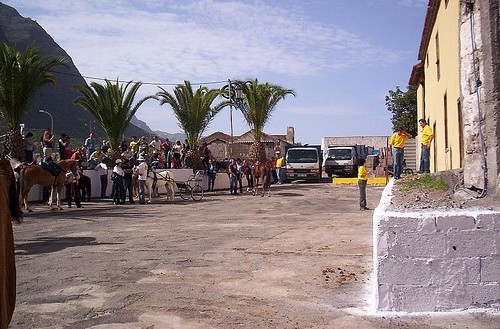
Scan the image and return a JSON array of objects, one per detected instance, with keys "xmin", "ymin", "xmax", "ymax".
[{"xmin": 131, "ymin": 161, "xmax": 204, "ymax": 203}]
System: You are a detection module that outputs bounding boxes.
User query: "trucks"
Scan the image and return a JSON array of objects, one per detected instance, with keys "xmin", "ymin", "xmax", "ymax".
[
  {"xmin": 283, "ymin": 145, "xmax": 324, "ymax": 184},
  {"xmin": 365, "ymin": 146, "xmax": 376, "ymax": 156},
  {"xmin": 323, "ymin": 144, "xmax": 367, "ymax": 182}
]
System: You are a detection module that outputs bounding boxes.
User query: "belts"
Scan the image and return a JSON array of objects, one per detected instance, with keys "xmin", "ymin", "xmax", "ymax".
[{"xmin": 394, "ymin": 147, "xmax": 404, "ymax": 149}]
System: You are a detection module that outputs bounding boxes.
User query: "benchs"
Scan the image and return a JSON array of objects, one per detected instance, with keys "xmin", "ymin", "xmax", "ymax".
[{"xmin": 188, "ymin": 170, "xmax": 204, "ymax": 181}]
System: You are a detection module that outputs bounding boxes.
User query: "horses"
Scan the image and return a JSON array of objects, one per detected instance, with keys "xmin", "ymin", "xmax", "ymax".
[
  {"xmin": 18, "ymin": 159, "xmax": 80, "ymax": 212},
  {"xmin": 132, "ymin": 165, "xmax": 177, "ymax": 204},
  {"xmin": 240, "ymin": 159, "xmax": 271, "ymax": 197}
]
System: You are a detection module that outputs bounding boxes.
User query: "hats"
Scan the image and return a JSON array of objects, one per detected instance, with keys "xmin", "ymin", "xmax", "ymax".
[
  {"xmin": 46, "ymin": 157, "xmax": 54, "ymax": 161},
  {"xmin": 116, "ymin": 159, "xmax": 122, "ymax": 163},
  {"xmin": 137, "ymin": 156, "xmax": 145, "ymax": 160}
]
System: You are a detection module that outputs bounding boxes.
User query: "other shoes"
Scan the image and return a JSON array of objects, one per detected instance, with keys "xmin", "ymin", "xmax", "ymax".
[
  {"xmin": 423, "ymin": 170, "xmax": 430, "ymax": 175},
  {"xmin": 138, "ymin": 202, "xmax": 145, "ymax": 205},
  {"xmin": 77, "ymin": 206, "xmax": 84, "ymax": 208},
  {"xmin": 360, "ymin": 207, "xmax": 364, "ymax": 211},
  {"xmin": 417, "ymin": 171, "xmax": 424, "ymax": 175},
  {"xmin": 112, "ymin": 201, "xmax": 115, "ymax": 205},
  {"xmin": 364, "ymin": 207, "xmax": 369, "ymax": 210},
  {"xmin": 117, "ymin": 202, "xmax": 123, "ymax": 205},
  {"xmin": 394, "ymin": 175, "xmax": 400, "ymax": 179},
  {"xmin": 68, "ymin": 205, "xmax": 71, "ymax": 208}
]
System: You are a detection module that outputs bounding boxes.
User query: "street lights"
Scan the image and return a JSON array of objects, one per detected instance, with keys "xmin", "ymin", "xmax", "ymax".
[
  {"xmin": 228, "ymin": 79, "xmax": 243, "ymax": 157},
  {"xmin": 38, "ymin": 109, "xmax": 53, "ymax": 132}
]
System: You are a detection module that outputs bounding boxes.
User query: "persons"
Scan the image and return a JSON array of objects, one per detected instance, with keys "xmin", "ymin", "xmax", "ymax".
[
  {"xmin": 120, "ymin": 134, "xmax": 190, "ymax": 169},
  {"xmin": 271, "ymin": 153, "xmax": 286, "ymax": 184},
  {"xmin": 229, "ymin": 154, "xmax": 243, "ymax": 194},
  {"xmin": 112, "ymin": 156, "xmax": 147, "ymax": 204},
  {"xmin": 416, "ymin": 119, "xmax": 433, "ymax": 173},
  {"xmin": 357, "ymin": 158, "xmax": 370, "ymax": 210},
  {"xmin": 390, "ymin": 127, "xmax": 412, "ymax": 179},
  {"xmin": 200, "ymin": 142, "xmax": 217, "ymax": 191},
  {"xmin": 25, "ymin": 130, "xmax": 108, "ymax": 208}
]
